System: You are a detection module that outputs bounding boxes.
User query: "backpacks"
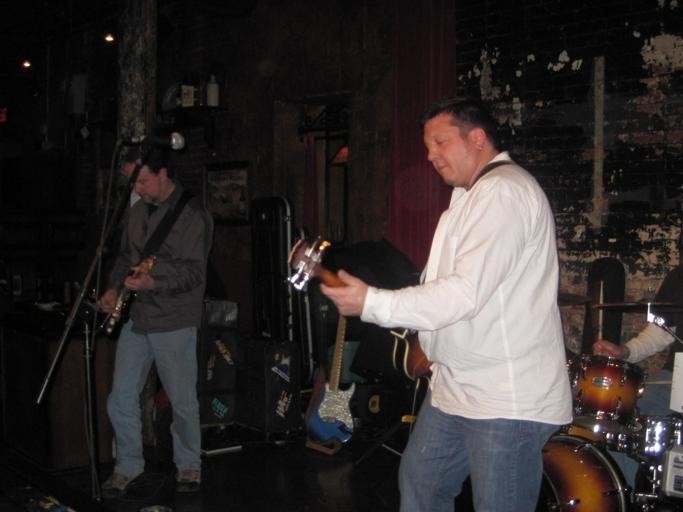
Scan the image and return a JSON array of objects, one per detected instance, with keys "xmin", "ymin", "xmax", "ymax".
[
  {"xmin": 620, "ymin": 414, "xmax": 683, "ymax": 463},
  {"xmin": 458, "ymin": 435, "xmax": 625, "ymax": 512},
  {"xmin": 565, "ymin": 354, "xmax": 643, "ymax": 433}
]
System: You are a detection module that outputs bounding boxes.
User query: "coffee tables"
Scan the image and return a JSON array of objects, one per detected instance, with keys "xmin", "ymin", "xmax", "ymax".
[
  {"xmin": 556, "ymin": 293, "xmax": 593, "ymax": 305},
  {"xmin": 595, "ymin": 300, "xmax": 683, "ymax": 313}
]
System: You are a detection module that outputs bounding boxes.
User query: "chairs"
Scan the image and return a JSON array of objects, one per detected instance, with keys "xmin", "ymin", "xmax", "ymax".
[
  {"xmin": 175, "ymin": 471, "xmax": 201, "ymax": 492},
  {"xmin": 108, "ymin": 470, "xmax": 132, "ymax": 493}
]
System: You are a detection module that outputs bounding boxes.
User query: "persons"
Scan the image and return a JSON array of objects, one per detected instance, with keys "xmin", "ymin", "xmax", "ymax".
[
  {"xmin": 318, "ymin": 99, "xmax": 573, "ymax": 512},
  {"xmin": 591, "ymin": 262, "xmax": 683, "ymax": 421},
  {"xmin": 98, "ymin": 142, "xmax": 215, "ymax": 499}
]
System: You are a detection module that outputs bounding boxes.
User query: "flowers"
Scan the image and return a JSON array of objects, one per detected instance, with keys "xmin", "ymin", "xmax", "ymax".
[{"xmin": 125, "ymin": 132, "xmax": 185, "ymax": 154}]
